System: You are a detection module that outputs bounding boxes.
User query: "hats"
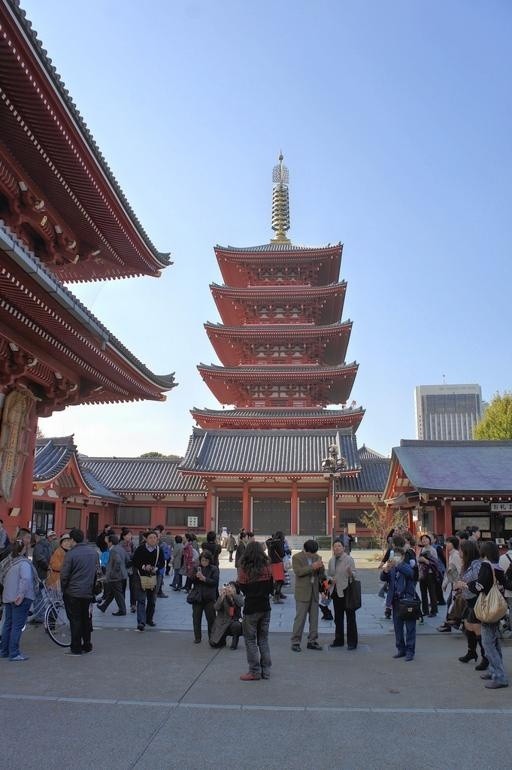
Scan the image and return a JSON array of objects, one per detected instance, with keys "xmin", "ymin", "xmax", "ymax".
[
  {"xmin": 47, "ymin": 531, "xmax": 56, "ymax": 537},
  {"xmin": 35, "ymin": 529, "xmax": 46, "ymax": 536},
  {"xmin": 60, "ymin": 534, "xmax": 70, "ymax": 541}
]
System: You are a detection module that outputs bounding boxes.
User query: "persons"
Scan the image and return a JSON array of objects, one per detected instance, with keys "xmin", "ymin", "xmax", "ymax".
[
  {"xmin": 378, "ymin": 529, "xmax": 462, "ymax": 662},
  {"xmin": 94, "ymin": 524, "xmax": 200, "ymax": 631},
  {"xmin": 190, "ymin": 526, "xmax": 292, "ymax": 680},
  {"xmin": 0, "ymin": 524, "xmax": 98, "ymax": 661},
  {"xmin": 453, "ymin": 525, "xmax": 510, "ymax": 688},
  {"xmin": 290, "ymin": 530, "xmax": 359, "ymax": 652}
]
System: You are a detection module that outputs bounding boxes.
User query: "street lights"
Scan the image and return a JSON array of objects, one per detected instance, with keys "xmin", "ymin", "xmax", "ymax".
[{"xmin": 318, "ymin": 441, "xmax": 346, "ymax": 542}]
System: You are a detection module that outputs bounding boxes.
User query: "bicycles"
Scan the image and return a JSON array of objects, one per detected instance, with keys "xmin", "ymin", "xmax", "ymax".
[{"xmin": 0, "ymin": 565, "xmax": 74, "ymax": 648}]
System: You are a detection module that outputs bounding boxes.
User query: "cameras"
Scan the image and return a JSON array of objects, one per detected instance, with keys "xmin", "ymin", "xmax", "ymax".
[{"xmin": 223, "ymin": 583, "xmax": 230, "ymax": 588}]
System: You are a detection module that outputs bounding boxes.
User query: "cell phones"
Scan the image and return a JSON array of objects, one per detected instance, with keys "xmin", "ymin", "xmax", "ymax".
[
  {"xmin": 197, "ymin": 566, "xmax": 202, "ymax": 573},
  {"xmin": 388, "ymin": 549, "xmax": 395, "ymax": 561}
]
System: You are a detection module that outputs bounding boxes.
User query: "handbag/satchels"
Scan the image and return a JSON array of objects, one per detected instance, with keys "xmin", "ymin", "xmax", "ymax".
[
  {"xmin": 282, "ymin": 554, "xmax": 290, "ymax": 572},
  {"xmin": 140, "ymin": 576, "xmax": 157, "ymax": 591}
]
